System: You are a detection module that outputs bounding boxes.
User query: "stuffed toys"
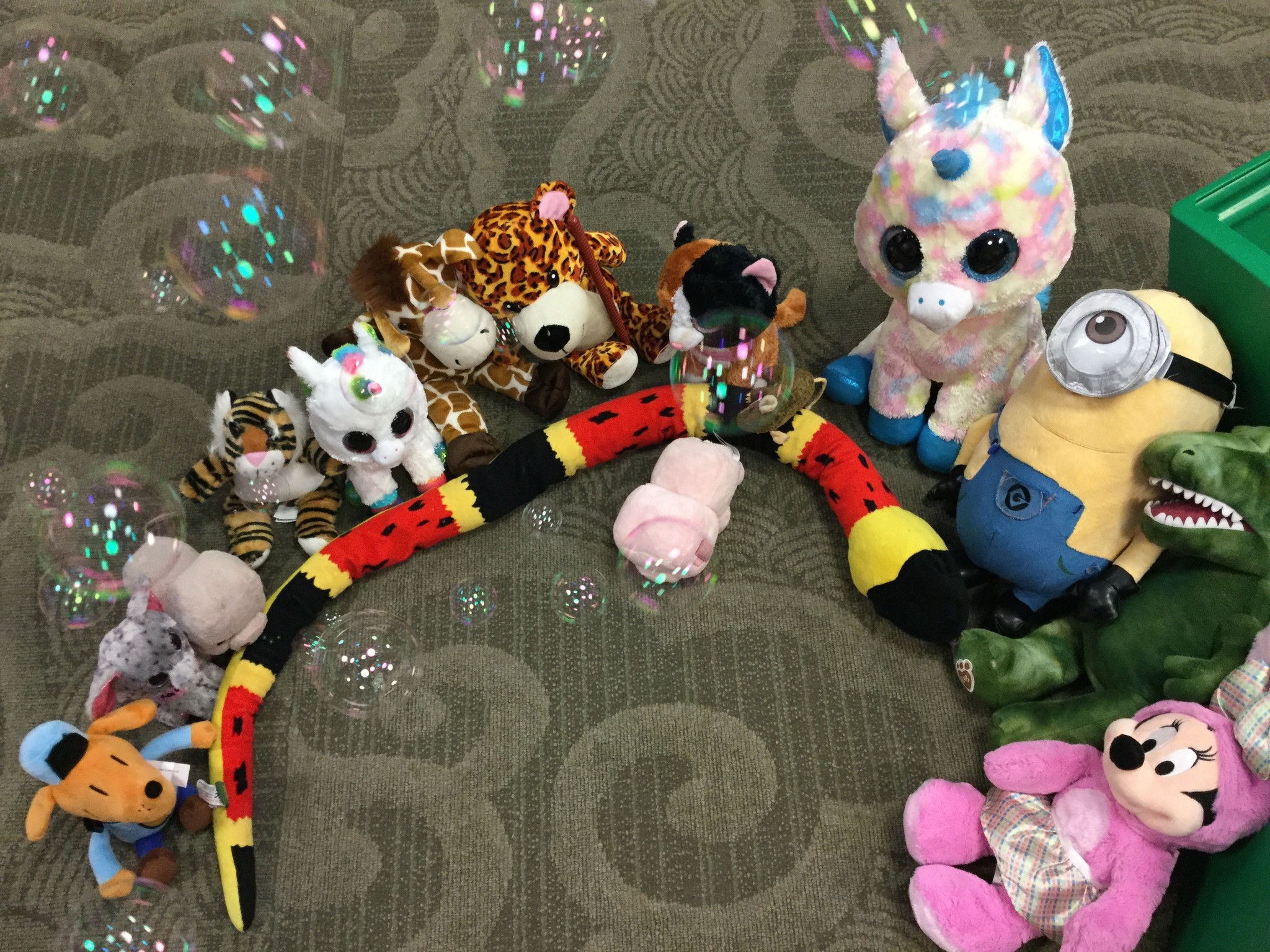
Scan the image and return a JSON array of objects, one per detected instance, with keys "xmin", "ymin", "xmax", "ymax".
[
  {"xmin": 207, "ymin": 384, "xmax": 999, "ymax": 932},
  {"xmin": 354, "ymin": 237, "xmax": 568, "ymax": 477},
  {"xmin": 464, "ymin": 184, "xmax": 678, "ymax": 387},
  {"xmin": 18, "ymin": 698, "xmax": 218, "ymax": 898},
  {"xmin": 613, "ymin": 437, "xmax": 745, "ymax": 583},
  {"xmin": 900, "ymin": 425, "xmax": 1270, "ymax": 952},
  {"xmin": 287, "ymin": 318, "xmax": 451, "ymax": 516},
  {"xmin": 922, "ymin": 287, "xmax": 1236, "ymax": 632},
  {"xmin": 82, "ymin": 590, "xmax": 226, "ymax": 729},
  {"xmin": 180, "ymin": 385, "xmax": 348, "ymax": 565},
  {"xmin": 823, "ymin": 36, "xmax": 1077, "ymax": 474},
  {"xmin": 653, "ymin": 219, "xmax": 808, "ymax": 386},
  {"xmin": 123, "ymin": 536, "xmax": 269, "ymax": 655}
]
